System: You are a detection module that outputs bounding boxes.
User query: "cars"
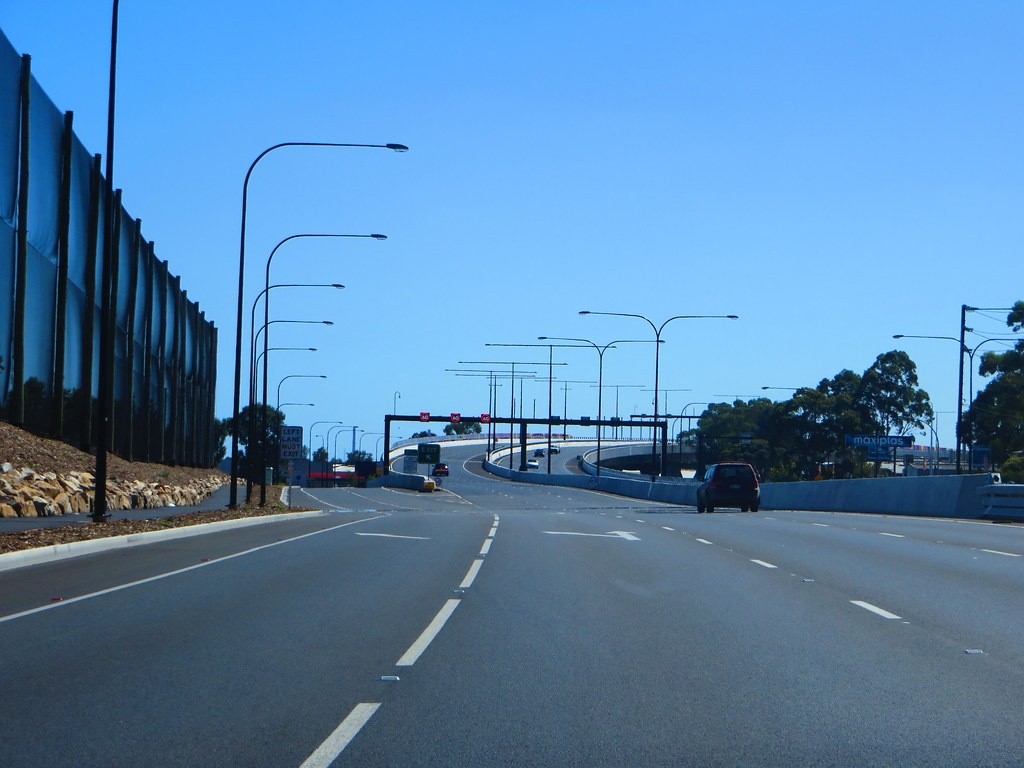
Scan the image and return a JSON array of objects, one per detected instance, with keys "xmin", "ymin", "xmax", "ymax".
[
  {"xmin": 534, "ymin": 448, "xmax": 546, "ymax": 457},
  {"xmin": 551, "ymin": 444, "xmax": 561, "ymax": 455},
  {"xmin": 526, "ymin": 459, "xmax": 539, "ymax": 469},
  {"xmin": 432, "ymin": 463, "xmax": 449, "ymax": 477},
  {"xmin": 697, "ymin": 460, "xmax": 760, "ymax": 514}
]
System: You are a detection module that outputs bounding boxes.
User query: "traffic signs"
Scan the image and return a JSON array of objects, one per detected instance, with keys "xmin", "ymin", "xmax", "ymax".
[{"xmin": 418, "ymin": 443, "xmax": 441, "ymax": 464}]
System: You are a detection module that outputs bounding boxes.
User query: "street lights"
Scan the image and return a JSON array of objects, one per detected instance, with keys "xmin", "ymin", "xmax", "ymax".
[
  {"xmin": 713, "ymin": 394, "xmax": 760, "ymax": 404},
  {"xmin": 454, "ymin": 373, "xmax": 535, "ymax": 450},
  {"xmin": 308, "ymin": 421, "xmax": 343, "ymax": 488},
  {"xmin": 244, "ymin": 320, "xmax": 333, "ymax": 504},
  {"xmin": 316, "ymin": 434, "xmax": 324, "ymax": 447},
  {"xmin": 458, "ymin": 362, "xmax": 569, "ymax": 470},
  {"xmin": 486, "ymin": 344, "xmax": 617, "ymax": 474},
  {"xmin": 892, "ymin": 335, "xmax": 1024, "ymax": 475},
  {"xmin": 254, "ymin": 347, "xmax": 317, "ymax": 502},
  {"xmin": 259, "ymin": 232, "xmax": 385, "ymax": 506},
  {"xmin": 640, "ymin": 389, "xmax": 692, "ymax": 422},
  {"xmin": 334, "ymin": 429, "xmax": 366, "ymax": 488},
  {"xmin": 580, "ymin": 311, "xmax": 739, "ymax": 482},
  {"xmin": 276, "ymin": 375, "xmax": 327, "ymax": 483},
  {"xmin": 760, "ymin": 386, "xmax": 853, "ymax": 482},
  {"xmin": 226, "ymin": 141, "xmax": 409, "ymax": 512},
  {"xmin": 589, "ymin": 385, "xmax": 645, "ymax": 438},
  {"xmin": 537, "ymin": 337, "xmax": 666, "ymax": 477},
  {"xmin": 486, "ymin": 377, "xmax": 557, "ymax": 445},
  {"xmin": 375, "ymin": 436, "xmax": 403, "ymax": 463},
  {"xmin": 393, "ymin": 391, "xmax": 401, "ymax": 415},
  {"xmin": 245, "ymin": 283, "xmax": 346, "ymax": 505},
  {"xmin": 536, "ymin": 379, "xmax": 597, "ymax": 440},
  {"xmin": 275, "ymin": 403, "xmax": 315, "ymax": 484},
  {"xmin": 445, "ymin": 369, "xmax": 537, "ymax": 461},
  {"xmin": 325, "ymin": 425, "xmax": 359, "ymax": 488}
]
